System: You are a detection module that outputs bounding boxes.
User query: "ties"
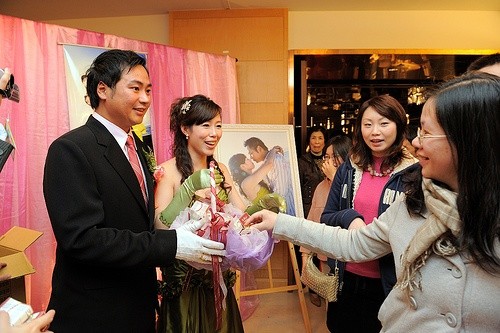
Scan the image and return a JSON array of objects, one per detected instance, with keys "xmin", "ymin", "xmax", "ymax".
[{"xmin": 126, "ymin": 135, "xmax": 148, "ymax": 211}]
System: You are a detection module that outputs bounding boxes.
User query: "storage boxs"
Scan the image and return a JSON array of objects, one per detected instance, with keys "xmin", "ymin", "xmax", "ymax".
[{"xmin": 0, "ymin": 225, "xmax": 45, "ymax": 304}]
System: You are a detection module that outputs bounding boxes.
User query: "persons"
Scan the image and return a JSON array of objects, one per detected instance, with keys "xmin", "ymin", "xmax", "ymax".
[
  {"xmin": 227, "ymin": 145, "xmax": 284, "ymax": 212},
  {"xmin": 243, "ymin": 70, "xmax": 500, "ymax": 333},
  {"xmin": 0, "ymin": 305, "xmax": 56, "ymax": 333},
  {"xmin": 296, "ymin": 124, "xmax": 329, "ymax": 306},
  {"xmin": 41, "ymin": 47, "xmax": 228, "ymax": 332},
  {"xmin": 244, "ymin": 136, "xmax": 297, "ymax": 218},
  {"xmin": 319, "ymin": 93, "xmax": 425, "ymax": 333},
  {"xmin": 297, "ymin": 134, "xmax": 353, "ymax": 284},
  {"xmin": 467, "ymin": 52, "xmax": 500, "ymax": 79},
  {"xmin": 399, "ymin": 124, "xmax": 418, "ymax": 159},
  {"xmin": 151, "ymin": 92, "xmax": 287, "ymax": 332}
]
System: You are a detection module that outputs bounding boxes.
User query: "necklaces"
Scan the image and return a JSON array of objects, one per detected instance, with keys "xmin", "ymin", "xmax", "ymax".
[{"xmin": 365, "ymin": 159, "xmax": 394, "ymax": 177}]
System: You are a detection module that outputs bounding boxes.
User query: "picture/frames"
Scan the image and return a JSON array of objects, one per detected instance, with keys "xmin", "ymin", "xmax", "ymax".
[{"xmin": 211, "ymin": 124, "xmax": 305, "ymax": 220}]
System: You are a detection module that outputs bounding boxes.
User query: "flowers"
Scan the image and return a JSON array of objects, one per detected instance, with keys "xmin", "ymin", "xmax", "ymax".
[{"xmin": 141, "ymin": 144, "xmax": 165, "ymax": 189}]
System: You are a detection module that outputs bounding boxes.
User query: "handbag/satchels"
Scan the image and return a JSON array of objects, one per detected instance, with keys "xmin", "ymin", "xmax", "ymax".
[{"xmin": 299, "ymin": 250, "xmax": 340, "ymax": 302}]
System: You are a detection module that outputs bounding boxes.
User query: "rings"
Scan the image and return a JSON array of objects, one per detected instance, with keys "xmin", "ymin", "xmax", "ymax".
[{"xmin": 200, "ymin": 252, "xmax": 204, "ymax": 260}]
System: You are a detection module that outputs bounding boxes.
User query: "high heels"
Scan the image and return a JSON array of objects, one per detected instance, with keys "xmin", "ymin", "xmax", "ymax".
[{"xmin": 309, "ymin": 293, "xmax": 322, "ymax": 307}]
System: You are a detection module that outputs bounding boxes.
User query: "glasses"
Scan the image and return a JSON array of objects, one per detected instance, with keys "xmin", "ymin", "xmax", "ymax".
[
  {"xmin": 322, "ymin": 154, "xmax": 341, "ymax": 161},
  {"xmin": 416, "ymin": 127, "xmax": 447, "ymax": 142}
]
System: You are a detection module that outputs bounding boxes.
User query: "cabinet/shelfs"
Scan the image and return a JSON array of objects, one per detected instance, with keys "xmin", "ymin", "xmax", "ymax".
[{"xmin": 306, "ymin": 76, "xmax": 435, "ymax": 142}]
